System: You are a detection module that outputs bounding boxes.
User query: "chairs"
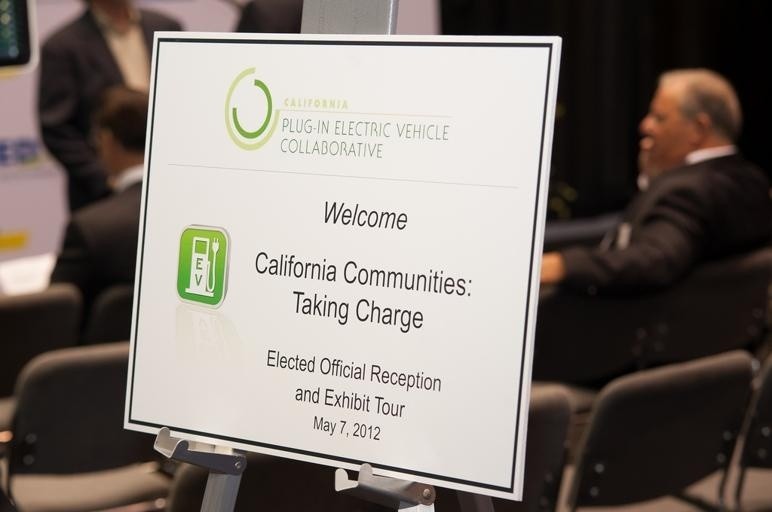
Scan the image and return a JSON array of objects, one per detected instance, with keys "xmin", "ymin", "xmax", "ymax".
[
  {"xmin": 733, "ymin": 353, "xmax": 772, "ymax": 511},
  {"xmin": 88, "ymin": 287, "xmax": 136, "ymax": 347},
  {"xmin": 532, "ymin": 246, "xmax": 772, "ymax": 385},
  {"xmin": 569, "ymin": 351, "xmax": 772, "ymax": 512},
  {"xmin": 0, "ymin": 342, "xmax": 166, "ymax": 510},
  {"xmin": 0, "ymin": 285, "xmax": 81, "ymax": 399},
  {"xmin": 169, "ymin": 457, "xmax": 357, "ymax": 510},
  {"xmin": 435, "ymin": 383, "xmax": 570, "ymax": 511}
]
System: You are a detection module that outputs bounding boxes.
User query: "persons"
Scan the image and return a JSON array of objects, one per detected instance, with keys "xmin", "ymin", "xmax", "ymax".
[
  {"xmin": 50, "ymin": 85, "xmax": 152, "ymax": 319},
  {"xmin": 539, "ymin": 67, "xmax": 768, "ymax": 392},
  {"xmin": 38, "ymin": 0, "xmax": 183, "ymax": 211}
]
